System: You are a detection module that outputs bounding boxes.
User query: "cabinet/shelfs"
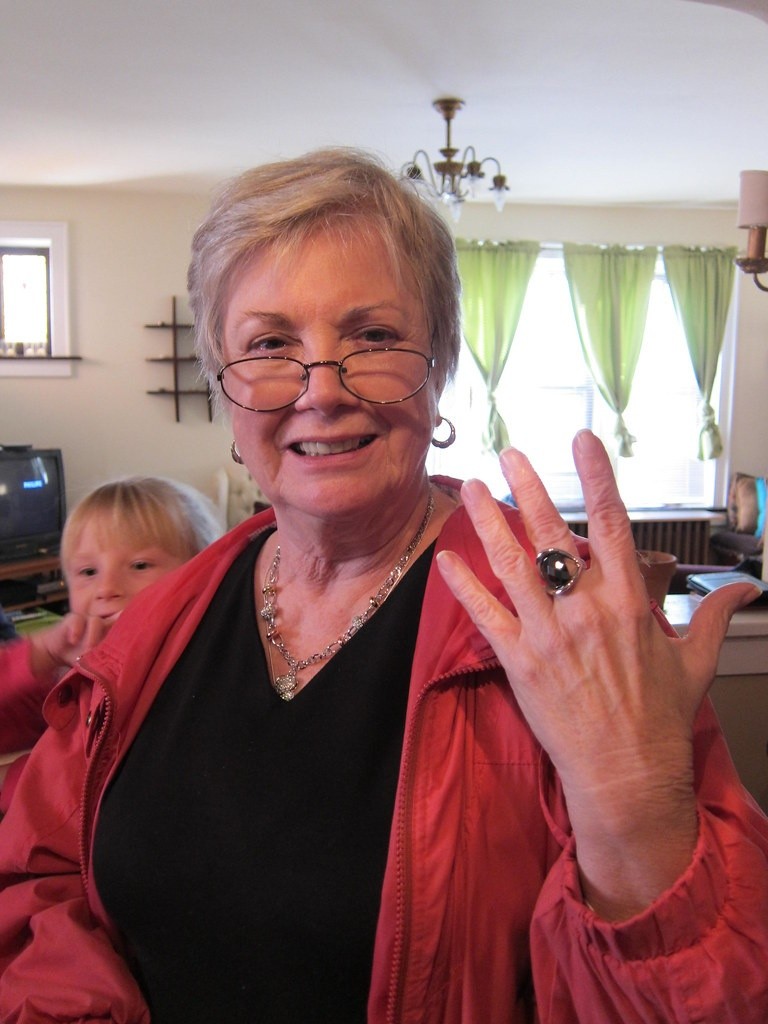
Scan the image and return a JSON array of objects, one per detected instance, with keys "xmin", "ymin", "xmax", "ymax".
[
  {"xmin": 144, "ymin": 296, "xmax": 218, "ymax": 423},
  {"xmin": 0, "ymin": 553, "xmax": 72, "ymax": 614}
]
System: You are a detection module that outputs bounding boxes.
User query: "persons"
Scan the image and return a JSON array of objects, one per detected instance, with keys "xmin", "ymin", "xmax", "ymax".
[
  {"xmin": 0, "ymin": 148, "xmax": 768, "ymax": 1023},
  {"xmin": 0, "ymin": 476, "xmax": 228, "ymax": 752}
]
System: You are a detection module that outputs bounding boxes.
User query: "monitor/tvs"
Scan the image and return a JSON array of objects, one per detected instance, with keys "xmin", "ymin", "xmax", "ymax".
[{"xmin": 0, "ymin": 449, "xmax": 67, "ymax": 563}]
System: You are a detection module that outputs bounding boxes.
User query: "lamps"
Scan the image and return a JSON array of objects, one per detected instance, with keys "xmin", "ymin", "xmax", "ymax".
[
  {"xmin": 731, "ymin": 170, "xmax": 768, "ymax": 293},
  {"xmin": 399, "ymin": 98, "xmax": 511, "ymax": 224}
]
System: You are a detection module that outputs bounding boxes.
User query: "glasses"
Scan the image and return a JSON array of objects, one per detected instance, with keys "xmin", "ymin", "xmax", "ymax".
[{"xmin": 216, "ymin": 328, "xmax": 438, "ymax": 412}]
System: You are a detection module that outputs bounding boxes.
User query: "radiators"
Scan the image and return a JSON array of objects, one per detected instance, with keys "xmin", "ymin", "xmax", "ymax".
[{"xmin": 569, "ymin": 521, "xmax": 710, "ymax": 564}]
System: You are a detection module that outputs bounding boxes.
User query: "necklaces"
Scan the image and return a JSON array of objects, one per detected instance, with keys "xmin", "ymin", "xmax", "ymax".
[{"xmin": 260, "ymin": 486, "xmax": 437, "ymax": 702}]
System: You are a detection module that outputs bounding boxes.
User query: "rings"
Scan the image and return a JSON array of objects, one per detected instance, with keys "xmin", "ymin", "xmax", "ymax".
[{"xmin": 536, "ymin": 547, "xmax": 588, "ymax": 597}]
